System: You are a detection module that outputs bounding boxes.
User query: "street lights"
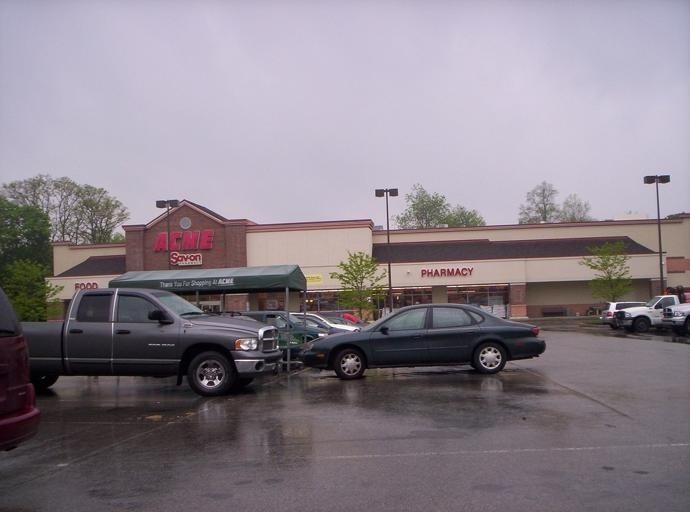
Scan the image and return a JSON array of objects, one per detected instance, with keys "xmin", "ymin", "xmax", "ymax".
[
  {"xmin": 644, "ymin": 175, "xmax": 670, "ymax": 295},
  {"xmin": 156, "ymin": 200, "xmax": 180, "ymax": 271},
  {"xmin": 375, "ymin": 189, "xmax": 398, "ymax": 313}
]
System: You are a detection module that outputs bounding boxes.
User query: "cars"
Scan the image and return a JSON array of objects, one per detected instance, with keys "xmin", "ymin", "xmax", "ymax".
[
  {"xmin": 239, "ymin": 309, "xmax": 373, "ymax": 372},
  {"xmin": 602, "ymin": 301, "xmax": 647, "ymax": 330},
  {"xmin": 300, "ymin": 303, "xmax": 545, "ymax": 380},
  {"xmin": 0, "ymin": 289, "xmax": 42, "ymax": 453},
  {"xmin": 662, "ymin": 302, "xmax": 690, "ymax": 336}
]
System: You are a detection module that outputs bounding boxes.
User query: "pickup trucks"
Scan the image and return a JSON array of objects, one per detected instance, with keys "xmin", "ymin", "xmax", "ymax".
[
  {"xmin": 17, "ymin": 288, "xmax": 284, "ymax": 397},
  {"xmin": 613, "ymin": 293, "xmax": 690, "ymax": 333}
]
